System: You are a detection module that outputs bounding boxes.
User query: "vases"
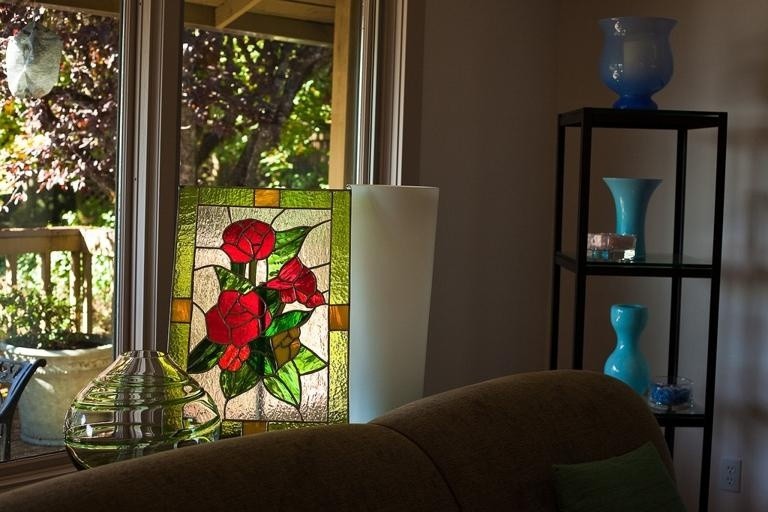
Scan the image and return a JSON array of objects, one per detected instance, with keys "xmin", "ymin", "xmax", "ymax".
[
  {"xmin": 63, "ymin": 347, "xmax": 226, "ymax": 471},
  {"xmin": 602, "ymin": 299, "xmax": 650, "ymax": 398},
  {"xmin": 601, "ymin": 177, "xmax": 664, "ymax": 258},
  {"xmin": 596, "ymin": 15, "xmax": 679, "ymax": 108}
]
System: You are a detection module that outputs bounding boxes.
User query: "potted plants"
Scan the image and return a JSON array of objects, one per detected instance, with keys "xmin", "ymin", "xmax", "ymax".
[{"xmin": 0, "ymin": 285, "xmax": 117, "ymax": 449}]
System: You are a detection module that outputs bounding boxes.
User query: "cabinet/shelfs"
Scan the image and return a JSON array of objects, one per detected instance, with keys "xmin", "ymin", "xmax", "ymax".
[{"xmin": 548, "ymin": 106, "xmax": 729, "ymax": 512}]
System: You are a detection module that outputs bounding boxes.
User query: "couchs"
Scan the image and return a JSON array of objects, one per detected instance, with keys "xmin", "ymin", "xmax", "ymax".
[{"xmin": 2, "ymin": 368, "xmax": 691, "ymax": 510}]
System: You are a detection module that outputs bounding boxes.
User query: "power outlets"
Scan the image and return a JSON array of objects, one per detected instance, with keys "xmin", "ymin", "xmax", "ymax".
[{"xmin": 717, "ymin": 452, "xmax": 742, "ymax": 493}]
